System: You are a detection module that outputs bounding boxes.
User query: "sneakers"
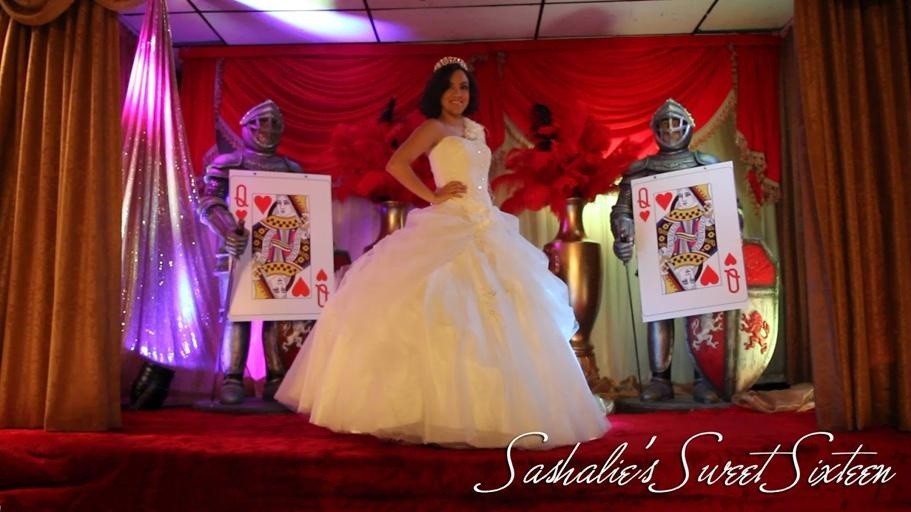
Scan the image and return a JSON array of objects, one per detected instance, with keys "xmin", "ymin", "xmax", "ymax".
[
  {"xmin": 693, "ymin": 378, "xmax": 717, "ymax": 403},
  {"xmin": 220, "ymin": 377, "xmax": 284, "ymax": 403},
  {"xmin": 642, "ymin": 377, "xmax": 673, "ymax": 402}
]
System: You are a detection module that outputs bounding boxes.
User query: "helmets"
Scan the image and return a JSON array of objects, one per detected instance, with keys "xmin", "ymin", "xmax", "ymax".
[
  {"xmin": 240, "ymin": 99, "xmax": 286, "ymax": 154},
  {"xmin": 650, "ymin": 98, "xmax": 696, "ymax": 153}
]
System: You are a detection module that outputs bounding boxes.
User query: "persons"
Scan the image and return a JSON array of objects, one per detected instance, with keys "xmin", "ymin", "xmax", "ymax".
[
  {"xmin": 195, "ymin": 99, "xmax": 302, "ymax": 404},
  {"xmin": 273, "ymin": 56, "xmax": 613, "ymax": 451},
  {"xmin": 609, "ymin": 98, "xmax": 745, "ymax": 404}
]
